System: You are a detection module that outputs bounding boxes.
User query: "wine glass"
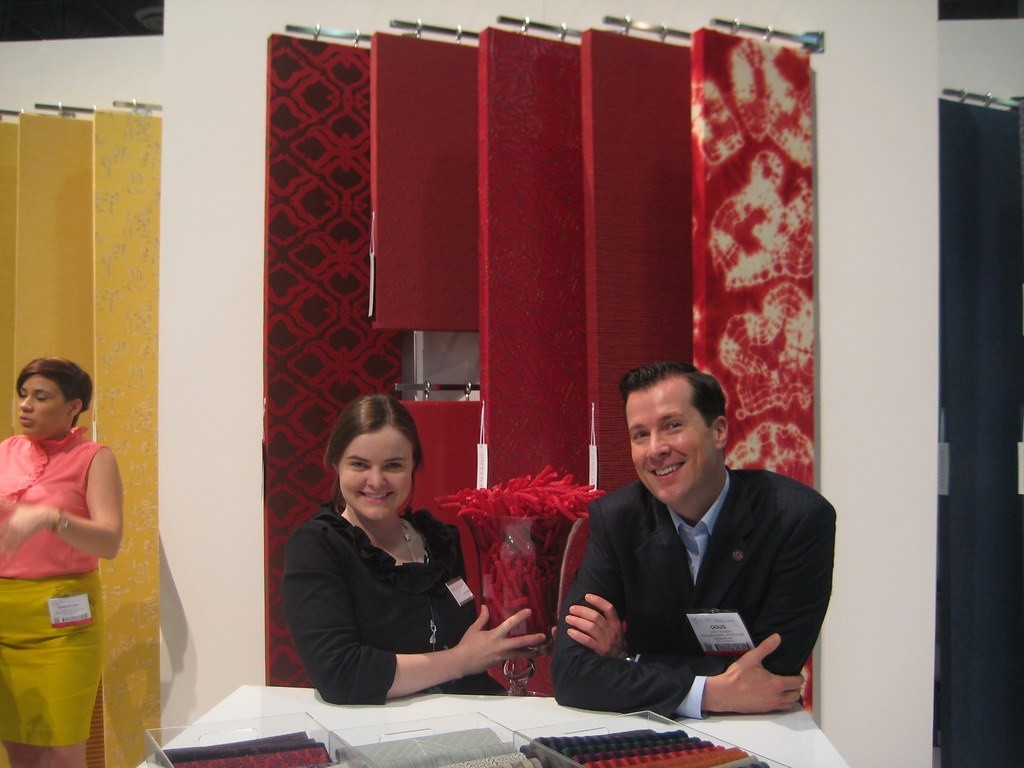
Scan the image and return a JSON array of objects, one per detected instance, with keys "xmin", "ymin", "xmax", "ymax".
[{"xmin": 461, "ymin": 511, "xmax": 574, "ymax": 697}]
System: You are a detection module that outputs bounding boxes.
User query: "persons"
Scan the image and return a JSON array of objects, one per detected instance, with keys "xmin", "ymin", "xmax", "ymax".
[
  {"xmin": 282, "ymin": 396, "xmax": 556, "ymax": 705},
  {"xmin": 0, "ymin": 358, "xmax": 124, "ymax": 768},
  {"xmin": 551, "ymin": 362, "xmax": 836, "ymax": 720}
]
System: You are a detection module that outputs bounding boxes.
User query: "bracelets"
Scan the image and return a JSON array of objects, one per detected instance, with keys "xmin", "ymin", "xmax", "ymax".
[
  {"xmin": 54, "ymin": 509, "xmax": 67, "ymax": 533},
  {"xmin": 625, "ymin": 655, "xmax": 634, "ymax": 662}
]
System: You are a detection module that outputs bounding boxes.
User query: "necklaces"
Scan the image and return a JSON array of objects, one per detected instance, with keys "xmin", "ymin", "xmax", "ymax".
[{"xmin": 402, "ymin": 519, "xmax": 416, "ymax": 563}]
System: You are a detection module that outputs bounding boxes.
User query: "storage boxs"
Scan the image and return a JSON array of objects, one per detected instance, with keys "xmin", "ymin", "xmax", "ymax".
[
  {"xmin": 513, "ymin": 710, "xmax": 793, "ymax": 768},
  {"xmin": 146, "ymin": 711, "xmax": 376, "ymax": 768},
  {"xmin": 332, "ymin": 711, "xmax": 584, "ymax": 768}
]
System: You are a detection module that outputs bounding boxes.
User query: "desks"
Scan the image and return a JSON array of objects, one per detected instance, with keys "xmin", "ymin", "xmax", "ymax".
[{"xmin": 135, "ymin": 685, "xmax": 847, "ymax": 768}]
